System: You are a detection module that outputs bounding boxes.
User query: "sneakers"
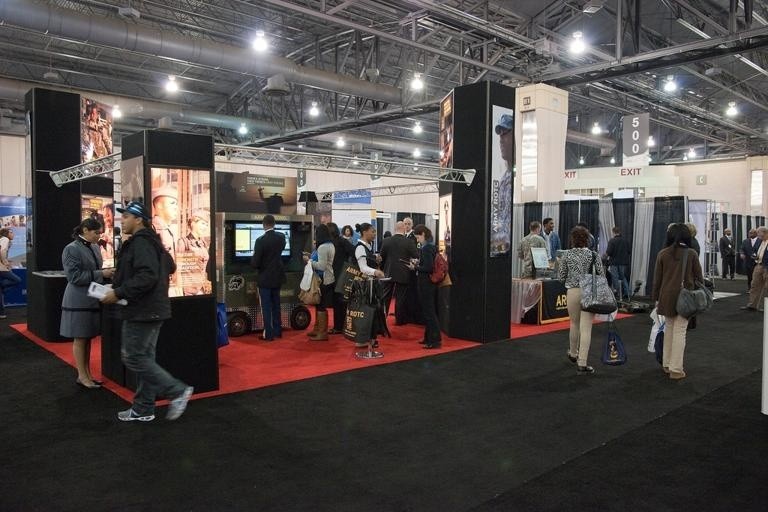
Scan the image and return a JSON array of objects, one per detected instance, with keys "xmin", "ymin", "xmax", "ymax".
[
  {"xmin": 566, "ymin": 350, "xmax": 578, "ymax": 365},
  {"xmin": 577, "ymin": 366, "xmax": 594, "ymax": 375},
  {"xmin": 165, "ymin": 386, "xmax": 194, "ymax": 422},
  {"xmin": 117, "ymin": 408, "xmax": 155, "ymax": 422}
]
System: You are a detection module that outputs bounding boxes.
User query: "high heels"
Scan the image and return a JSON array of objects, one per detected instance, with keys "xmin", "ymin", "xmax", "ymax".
[{"xmin": 670, "ymin": 372, "xmax": 686, "ymax": 379}]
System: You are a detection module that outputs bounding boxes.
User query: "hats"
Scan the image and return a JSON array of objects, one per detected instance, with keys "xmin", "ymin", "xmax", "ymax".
[{"xmin": 116, "ymin": 201, "xmax": 148, "ymax": 221}]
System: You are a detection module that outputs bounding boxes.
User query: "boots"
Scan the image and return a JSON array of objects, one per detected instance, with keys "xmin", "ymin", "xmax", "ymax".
[{"xmin": 308, "ymin": 310, "xmax": 328, "ymax": 341}]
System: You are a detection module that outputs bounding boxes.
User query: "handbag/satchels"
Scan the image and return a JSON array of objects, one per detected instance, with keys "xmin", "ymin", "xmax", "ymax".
[
  {"xmin": 297, "ymin": 261, "xmax": 323, "ymax": 305},
  {"xmin": 577, "ymin": 251, "xmax": 617, "ymax": 313},
  {"xmin": 602, "ymin": 315, "xmax": 627, "ymax": 366},
  {"xmin": 674, "ymin": 288, "xmax": 709, "ymax": 317},
  {"xmin": 654, "ymin": 327, "xmax": 663, "ymax": 365}
]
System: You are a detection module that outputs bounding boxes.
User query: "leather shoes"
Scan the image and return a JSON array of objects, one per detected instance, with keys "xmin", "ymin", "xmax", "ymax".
[
  {"xmin": 422, "ymin": 343, "xmax": 441, "ymax": 348},
  {"xmin": 76, "ymin": 377, "xmax": 104, "ymax": 389},
  {"xmin": 740, "ymin": 306, "xmax": 756, "ymax": 311}
]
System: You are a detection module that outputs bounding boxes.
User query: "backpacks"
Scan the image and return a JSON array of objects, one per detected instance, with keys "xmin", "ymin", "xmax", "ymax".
[{"xmin": 427, "ymin": 245, "xmax": 448, "ymax": 284}]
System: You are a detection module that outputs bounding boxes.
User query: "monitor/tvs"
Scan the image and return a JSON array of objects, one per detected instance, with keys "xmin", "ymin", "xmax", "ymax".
[{"xmin": 234, "ymin": 221, "xmax": 293, "ymax": 262}]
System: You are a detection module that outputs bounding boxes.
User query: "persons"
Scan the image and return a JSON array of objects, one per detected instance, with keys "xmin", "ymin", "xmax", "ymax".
[
  {"xmin": 60, "ymin": 218, "xmax": 116, "ymax": 388},
  {"xmin": 557, "ymin": 225, "xmax": 605, "ymax": 375},
  {"xmin": 315, "ymin": 203, "xmax": 331, "ymax": 226},
  {"xmin": 720, "ymin": 228, "xmax": 736, "ymax": 281},
  {"xmin": 1, "ymin": 215, "xmax": 25, "ymax": 227},
  {"xmin": 249, "ymin": 215, "xmax": 286, "ymax": 341},
  {"xmin": 177, "ymin": 210, "xmax": 212, "ymax": 296},
  {"xmin": 114, "ymin": 227, "xmax": 123, "ymax": 256},
  {"xmin": 576, "ymin": 222, "xmax": 595, "ymax": 252},
  {"xmin": 302, "ymin": 217, "xmax": 441, "ymax": 359},
  {"xmin": 219, "ymin": 174, "xmax": 237, "ymax": 211},
  {"xmin": 602, "ymin": 227, "xmax": 631, "ymax": 300},
  {"xmin": 98, "ymin": 201, "xmax": 195, "ymax": 423},
  {"xmin": 152, "ymin": 188, "xmax": 183, "ymax": 297},
  {"xmin": 651, "ymin": 223, "xmax": 704, "ymax": 379},
  {"xmin": 539, "ymin": 218, "xmax": 561, "ymax": 261},
  {"xmin": 518, "ymin": 222, "xmax": 545, "ymax": 280},
  {"xmin": 0, "ymin": 228, "xmax": 22, "ymax": 319},
  {"xmin": 100, "ymin": 204, "xmax": 114, "ymax": 259},
  {"xmin": 488, "ymin": 115, "xmax": 515, "ymax": 257},
  {"xmin": 739, "ymin": 226, "xmax": 768, "ymax": 312},
  {"xmin": 81, "ymin": 103, "xmax": 112, "ymax": 160},
  {"xmin": 740, "ymin": 227, "xmax": 762, "ymax": 294}
]
